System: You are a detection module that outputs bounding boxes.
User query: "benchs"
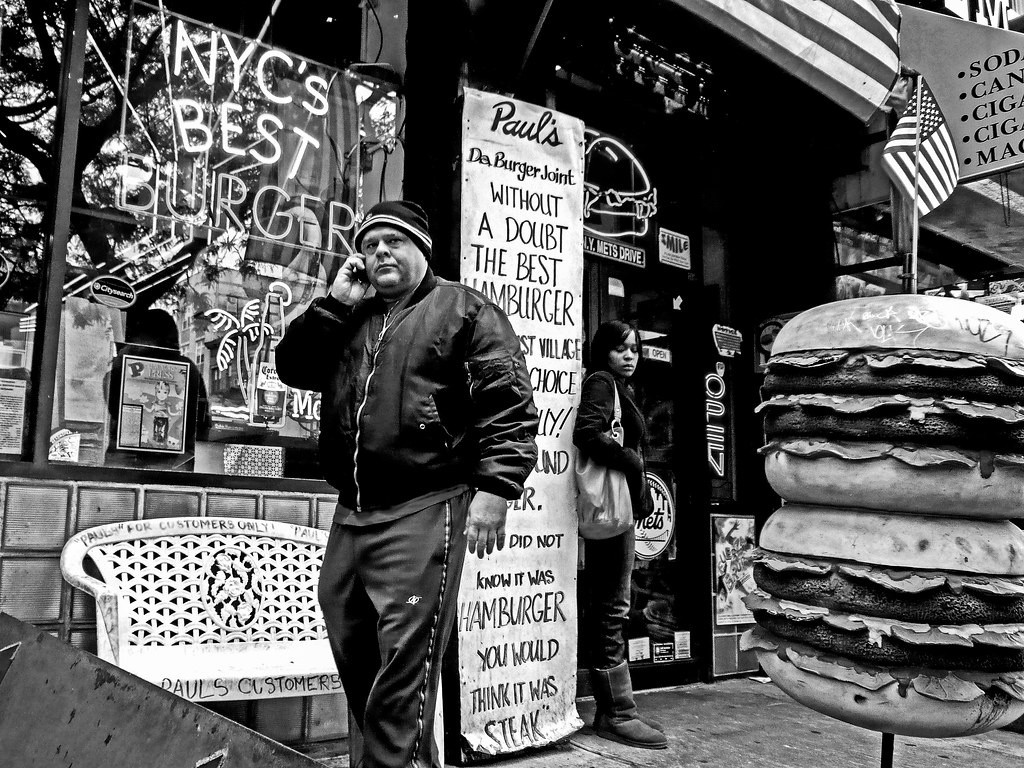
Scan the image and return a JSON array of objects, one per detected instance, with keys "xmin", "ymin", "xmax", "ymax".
[{"xmin": 60, "ymin": 518, "xmax": 444, "ymax": 768}]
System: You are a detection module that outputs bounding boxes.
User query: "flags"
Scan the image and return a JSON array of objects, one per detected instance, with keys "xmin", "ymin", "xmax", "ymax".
[
  {"xmin": 880, "ymin": 74, "xmax": 961, "ymax": 219},
  {"xmin": 671, "ymin": 0, "xmax": 900, "ymax": 123}
]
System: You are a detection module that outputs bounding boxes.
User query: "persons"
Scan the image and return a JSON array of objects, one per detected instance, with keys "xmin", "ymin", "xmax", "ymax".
[
  {"xmin": 573, "ymin": 320, "xmax": 671, "ymax": 752},
  {"xmin": 273, "ymin": 198, "xmax": 539, "ymax": 768}
]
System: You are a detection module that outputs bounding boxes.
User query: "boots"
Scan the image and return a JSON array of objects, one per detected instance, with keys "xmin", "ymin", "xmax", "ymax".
[{"xmin": 588, "ymin": 660, "xmax": 669, "ymax": 749}]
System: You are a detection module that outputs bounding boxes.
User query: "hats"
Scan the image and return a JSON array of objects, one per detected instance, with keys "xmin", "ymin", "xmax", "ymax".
[{"xmin": 354, "ymin": 200, "xmax": 433, "ymax": 262}]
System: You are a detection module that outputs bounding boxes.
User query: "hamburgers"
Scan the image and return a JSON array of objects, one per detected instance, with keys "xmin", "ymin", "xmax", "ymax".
[{"xmin": 738, "ymin": 293, "xmax": 1024, "ymax": 738}]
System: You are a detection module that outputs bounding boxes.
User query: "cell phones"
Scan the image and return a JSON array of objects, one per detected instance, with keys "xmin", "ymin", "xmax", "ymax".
[{"xmin": 353, "ymin": 259, "xmax": 367, "ymax": 284}]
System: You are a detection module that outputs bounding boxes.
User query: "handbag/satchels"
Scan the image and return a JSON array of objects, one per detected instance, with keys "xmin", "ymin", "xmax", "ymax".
[{"xmin": 574, "ymin": 371, "xmax": 633, "ymax": 541}]
